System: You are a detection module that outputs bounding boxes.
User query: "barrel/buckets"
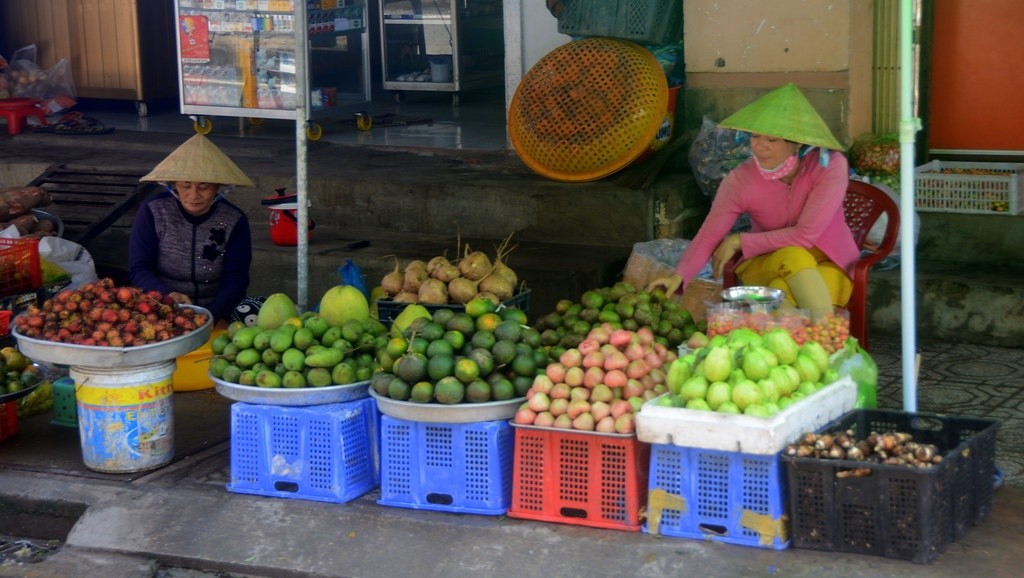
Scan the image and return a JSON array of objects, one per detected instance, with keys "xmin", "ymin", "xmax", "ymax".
[
  {"xmin": 69, "ymin": 357, "xmax": 175, "ymax": 474},
  {"xmin": 261, "ymin": 187, "xmax": 316, "ymax": 246}
]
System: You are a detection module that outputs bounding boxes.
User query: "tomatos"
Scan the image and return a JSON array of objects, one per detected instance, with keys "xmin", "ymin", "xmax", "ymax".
[
  {"xmin": 706, "ymin": 313, "xmax": 849, "ymax": 354},
  {"xmin": 852, "ymin": 142, "xmax": 901, "ymax": 173}
]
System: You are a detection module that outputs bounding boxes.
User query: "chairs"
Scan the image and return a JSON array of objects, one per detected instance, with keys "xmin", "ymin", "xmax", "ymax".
[{"xmin": 723, "ymin": 178, "xmax": 901, "ymax": 349}]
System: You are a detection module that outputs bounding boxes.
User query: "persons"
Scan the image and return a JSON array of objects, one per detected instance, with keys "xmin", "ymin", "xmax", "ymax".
[
  {"xmin": 127, "ymin": 133, "xmax": 256, "ymax": 327},
  {"xmin": 646, "ymin": 83, "xmax": 860, "ymax": 327}
]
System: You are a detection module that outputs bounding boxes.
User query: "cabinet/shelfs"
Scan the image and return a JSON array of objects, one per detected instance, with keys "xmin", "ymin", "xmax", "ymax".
[
  {"xmin": 378, "ymin": 0, "xmax": 503, "ymax": 106},
  {"xmin": 0, "ymin": 0, "xmax": 179, "ymax": 117},
  {"xmin": 173, "ymin": 0, "xmax": 372, "ymax": 141}
]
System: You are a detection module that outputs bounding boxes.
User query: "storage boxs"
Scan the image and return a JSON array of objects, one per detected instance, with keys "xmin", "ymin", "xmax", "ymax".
[
  {"xmin": 507, "ymin": 429, "xmax": 650, "ymax": 533},
  {"xmin": 378, "ymin": 286, "xmax": 531, "ymax": 333},
  {"xmin": 625, "ymin": 239, "xmax": 723, "ymax": 325},
  {"xmin": 642, "ymin": 444, "xmax": 792, "ymax": 552},
  {"xmin": 914, "ymin": 160, "xmax": 1024, "ymax": 216},
  {"xmin": 634, "ymin": 377, "xmax": 859, "ymax": 455},
  {"xmin": 0, "ymin": 237, "xmax": 41, "ymax": 299},
  {"xmin": 379, "ymin": 414, "xmax": 516, "ymax": 515},
  {"xmin": 223, "ymin": 400, "xmax": 383, "ymax": 504},
  {"xmin": 782, "ymin": 408, "xmax": 1000, "ymax": 563}
]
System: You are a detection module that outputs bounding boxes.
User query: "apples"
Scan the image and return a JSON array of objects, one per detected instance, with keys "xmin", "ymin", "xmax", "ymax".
[{"xmin": 514, "ymin": 328, "xmax": 677, "ymax": 434}]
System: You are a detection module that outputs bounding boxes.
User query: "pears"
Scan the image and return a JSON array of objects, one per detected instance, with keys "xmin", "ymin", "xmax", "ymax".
[
  {"xmin": 666, "ymin": 330, "xmax": 839, "ymax": 417},
  {"xmin": 211, "ymin": 321, "xmax": 386, "ymax": 389}
]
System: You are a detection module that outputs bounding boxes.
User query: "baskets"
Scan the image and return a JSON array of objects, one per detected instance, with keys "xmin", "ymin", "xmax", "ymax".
[{"xmin": 913, "ymin": 160, "xmax": 1024, "ymax": 216}]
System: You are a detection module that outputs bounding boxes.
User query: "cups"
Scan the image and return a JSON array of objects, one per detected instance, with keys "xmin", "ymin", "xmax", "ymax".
[{"xmin": 431, "ymin": 64, "xmax": 449, "ymax": 81}]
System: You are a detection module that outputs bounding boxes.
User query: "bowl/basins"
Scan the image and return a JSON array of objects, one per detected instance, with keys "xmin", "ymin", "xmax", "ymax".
[{"xmin": 721, "ymin": 286, "xmax": 787, "ymax": 304}]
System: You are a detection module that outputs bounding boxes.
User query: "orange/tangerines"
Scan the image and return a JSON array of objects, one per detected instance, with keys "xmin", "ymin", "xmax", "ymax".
[
  {"xmin": 0, "ymin": 347, "xmax": 42, "ymax": 395},
  {"xmin": 371, "ymin": 298, "xmax": 566, "ymax": 404}
]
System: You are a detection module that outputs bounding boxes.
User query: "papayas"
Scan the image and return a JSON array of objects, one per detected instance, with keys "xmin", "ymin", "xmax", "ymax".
[
  {"xmin": 390, "ymin": 303, "xmax": 434, "ymax": 340},
  {"xmin": 257, "ymin": 293, "xmax": 298, "ymax": 330},
  {"xmin": 319, "ymin": 285, "xmax": 369, "ymax": 326}
]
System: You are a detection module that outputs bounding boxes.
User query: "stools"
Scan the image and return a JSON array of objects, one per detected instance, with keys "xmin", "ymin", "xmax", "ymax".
[{"xmin": 0, "ymin": 98, "xmax": 46, "ymax": 135}]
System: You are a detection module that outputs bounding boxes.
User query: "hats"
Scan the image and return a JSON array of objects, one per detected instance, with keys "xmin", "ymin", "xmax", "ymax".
[
  {"xmin": 139, "ymin": 130, "xmax": 255, "ymax": 187},
  {"xmin": 715, "ymin": 82, "xmax": 848, "ymax": 153}
]
